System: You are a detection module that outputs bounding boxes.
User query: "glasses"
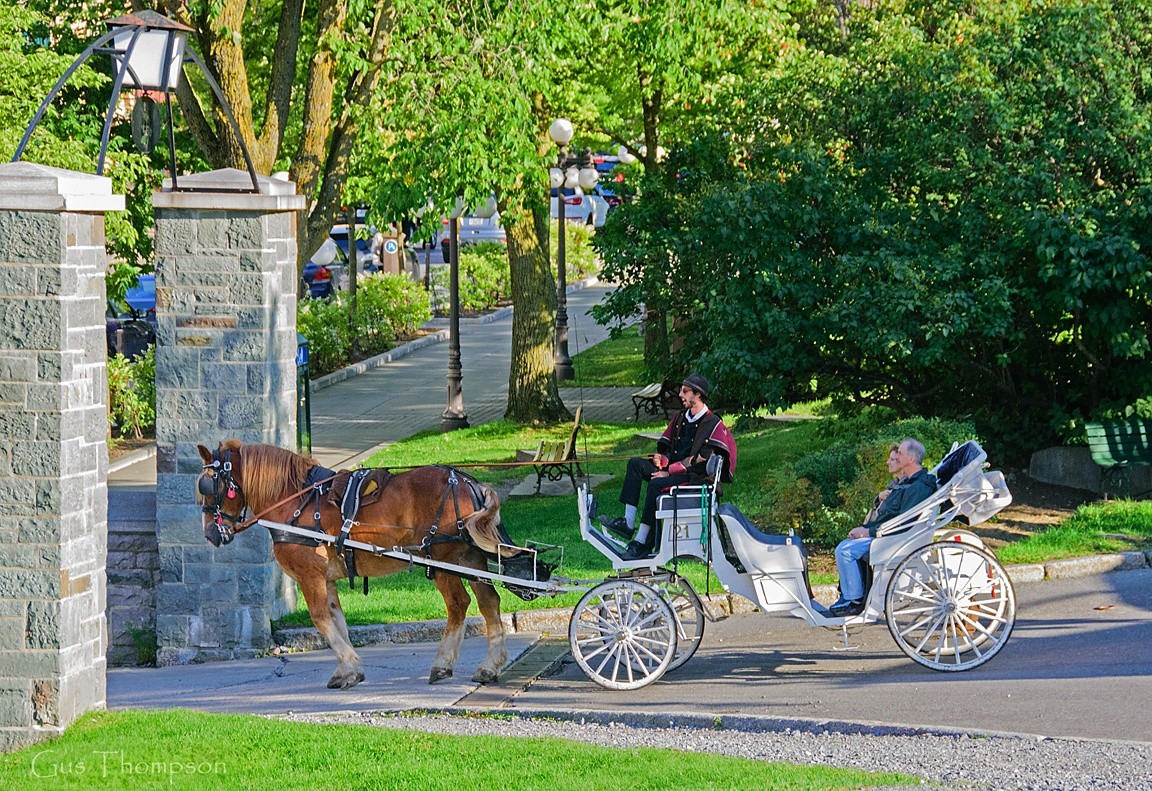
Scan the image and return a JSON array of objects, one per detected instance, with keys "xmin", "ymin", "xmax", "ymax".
[{"xmin": 679, "ymin": 388, "xmax": 693, "ymax": 394}]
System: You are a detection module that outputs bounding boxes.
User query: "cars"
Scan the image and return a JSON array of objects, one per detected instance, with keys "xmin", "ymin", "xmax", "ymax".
[
  {"xmin": 304, "ymin": 237, "xmax": 351, "ymax": 299},
  {"xmin": 330, "ymin": 224, "xmax": 421, "ymax": 284},
  {"xmin": 590, "ymin": 156, "xmax": 625, "ymax": 208},
  {"xmin": 550, "ymin": 186, "xmax": 610, "ymax": 229},
  {"xmin": 340, "ymin": 202, "xmax": 438, "ymax": 251},
  {"xmin": 440, "ymin": 196, "xmax": 507, "ymax": 262},
  {"xmin": 106, "ymin": 291, "xmax": 155, "ymax": 362},
  {"xmin": 122, "ymin": 275, "xmax": 157, "ymax": 331}
]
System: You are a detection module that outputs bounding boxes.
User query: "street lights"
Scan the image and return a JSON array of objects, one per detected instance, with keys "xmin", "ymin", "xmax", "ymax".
[
  {"xmin": 549, "ymin": 119, "xmax": 599, "ymax": 382},
  {"xmin": 410, "ymin": 187, "xmax": 497, "ymax": 433}
]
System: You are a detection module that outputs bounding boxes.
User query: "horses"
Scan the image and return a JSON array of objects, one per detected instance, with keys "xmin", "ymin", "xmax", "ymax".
[{"xmin": 196, "ymin": 440, "xmax": 525, "ymax": 688}]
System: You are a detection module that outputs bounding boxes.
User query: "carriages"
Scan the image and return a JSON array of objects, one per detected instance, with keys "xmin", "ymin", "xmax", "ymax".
[{"xmin": 196, "ymin": 440, "xmax": 1018, "ymax": 693}]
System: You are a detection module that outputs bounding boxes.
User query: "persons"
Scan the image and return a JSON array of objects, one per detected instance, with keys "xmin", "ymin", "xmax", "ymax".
[
  {"xmin": 830, "ymin": 437, "xmax": 937, "ymax": 617},
  {"xmin": 371, "ymin": 222, "xmax": 393, "ymax": 271},
  {"xmin": 601, "ymin": 374, "xmax": 737, "ymax": 561}
]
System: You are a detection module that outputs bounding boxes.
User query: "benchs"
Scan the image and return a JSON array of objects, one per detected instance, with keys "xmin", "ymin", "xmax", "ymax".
[
  {"xmin": 862, "ymin": 495, "xmax": 939, "ymax": 565},
  {"xmin": 630, "ymin": 379, "xmax": 685, "ymax": 423},
  {"xmin": 655, "ymin": 455, "xmax": 724, "ymax": 520},
  {"xmin": 716, "ymin": 504, "xmax": 809, "ymax": 575},
  {"xmin": 1084, "ymin": 417, "xmax": 1152, "ymax": 498},
  {"xmin": 532, "ymin": 404, "xmax": 583, "ymax": 495}
]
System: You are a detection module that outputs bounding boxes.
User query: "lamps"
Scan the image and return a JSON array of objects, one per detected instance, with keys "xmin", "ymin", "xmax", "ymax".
[{"xmin": 106, "ymin": 10, "xmax": 197, "ymax": 95}]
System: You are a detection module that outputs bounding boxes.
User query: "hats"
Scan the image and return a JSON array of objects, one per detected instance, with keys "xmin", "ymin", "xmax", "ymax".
[{"xmin": 682, "ymin": 375, "xmax": 714, "ymax": 402}]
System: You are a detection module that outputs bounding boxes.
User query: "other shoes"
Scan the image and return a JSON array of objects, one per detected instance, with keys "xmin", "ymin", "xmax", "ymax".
[
  {"xmin": 834, "ymin": 601, "xmax": 863, "ymax": 616},
  {"xmin": 598, "ymin": 515, "xmax": 638, "ymax": 540},
  {"xmin": 829, "ymin": 594, "xmax": 851, "ymax": 612},
  {"xmin": 620, "ymin": 540, "xmax": 649, "ymax": 560}
]
